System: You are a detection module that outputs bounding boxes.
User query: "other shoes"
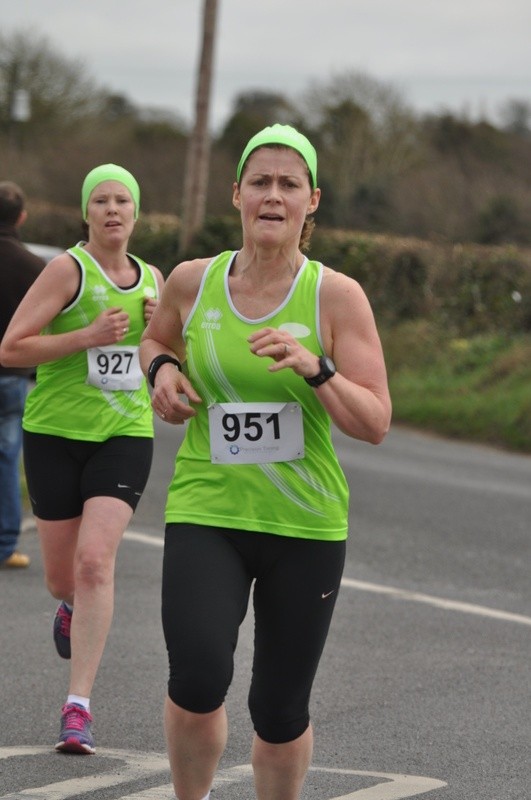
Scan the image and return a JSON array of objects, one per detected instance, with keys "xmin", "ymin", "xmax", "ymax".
[{"xmin": 2, "ymin": 552, "xmax": 29, "ymax": 569}]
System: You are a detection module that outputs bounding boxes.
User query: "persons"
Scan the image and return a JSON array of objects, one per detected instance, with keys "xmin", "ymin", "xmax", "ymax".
[
  {"xmin": 140, "ymin": 123, "xmax": 393, "ymax": 800},
  {"xmin": 0, "ymin": 164, "xmax": 166, "ymax": 755},
  {"xmin": 0, "ymin": 183, "xmax": 47, "ymax": 569}
]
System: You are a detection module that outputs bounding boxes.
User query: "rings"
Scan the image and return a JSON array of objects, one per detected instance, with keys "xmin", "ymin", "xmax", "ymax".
[
  {"xmin": 123, "ymin": 328, "xmax": 126, "ymax": 333},
  {"xmin": 285, "ymin": 343, "xmax": 288, "ymax": 353}
]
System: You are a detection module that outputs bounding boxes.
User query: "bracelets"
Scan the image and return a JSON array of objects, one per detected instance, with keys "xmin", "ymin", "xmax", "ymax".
[{"xmin": 147, "ymin": 353, "xmax": 182, "ymax": 390}]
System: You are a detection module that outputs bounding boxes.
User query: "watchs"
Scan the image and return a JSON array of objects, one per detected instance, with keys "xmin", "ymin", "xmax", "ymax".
[{"xmin": 304, "ymin": 355, "xmax": 336, "ymax": 388}]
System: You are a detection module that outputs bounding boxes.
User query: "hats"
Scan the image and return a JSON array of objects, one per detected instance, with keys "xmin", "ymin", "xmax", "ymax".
[
  {"xmin": 82, "ymin": 164, "xmax": 140, "ymax": 219},
  {"xmin": 237, "ymin": 123, "xmax": 317, "ymax": 190}
]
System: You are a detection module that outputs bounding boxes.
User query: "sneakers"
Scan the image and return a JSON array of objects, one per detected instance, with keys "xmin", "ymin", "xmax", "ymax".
[
  {"xmin": 52, "ymin": 600, "xmax": 74, "ymax": 659},
  {"xmin": 56, "ymin": 702, "xmax": 96, "ymax": 755}
]
